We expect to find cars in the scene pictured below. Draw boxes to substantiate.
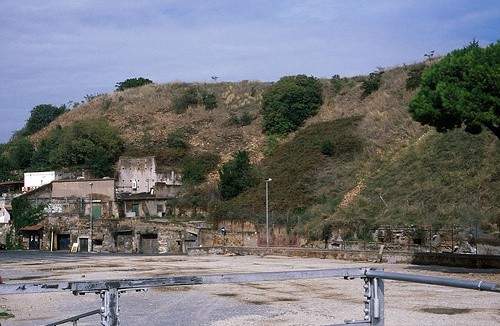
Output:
[76,177,84,180]
[103,177,110,179]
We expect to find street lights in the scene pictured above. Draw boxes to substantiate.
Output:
[89,183,94,251]
[265,178,272,249]
